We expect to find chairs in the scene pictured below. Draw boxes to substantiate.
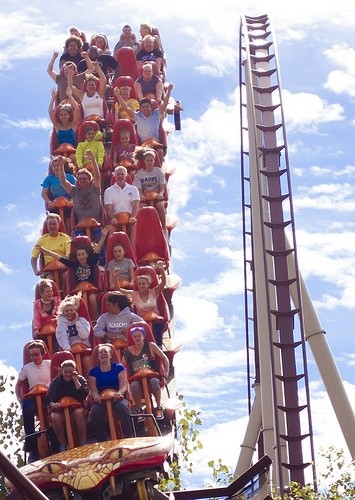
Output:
[13,30,181,425]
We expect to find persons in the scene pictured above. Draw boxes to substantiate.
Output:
[120,326,170,420]
[15,343,52,464]
[85,346,133,438]
[31,23,174,364]
[46,359,89,452]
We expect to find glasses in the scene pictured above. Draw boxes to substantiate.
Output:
[78,177,89,181]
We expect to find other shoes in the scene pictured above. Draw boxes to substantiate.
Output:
[155,406,164,420]
[136,408,145,422]
[162,229,168,241]
[28,451,37,463]
